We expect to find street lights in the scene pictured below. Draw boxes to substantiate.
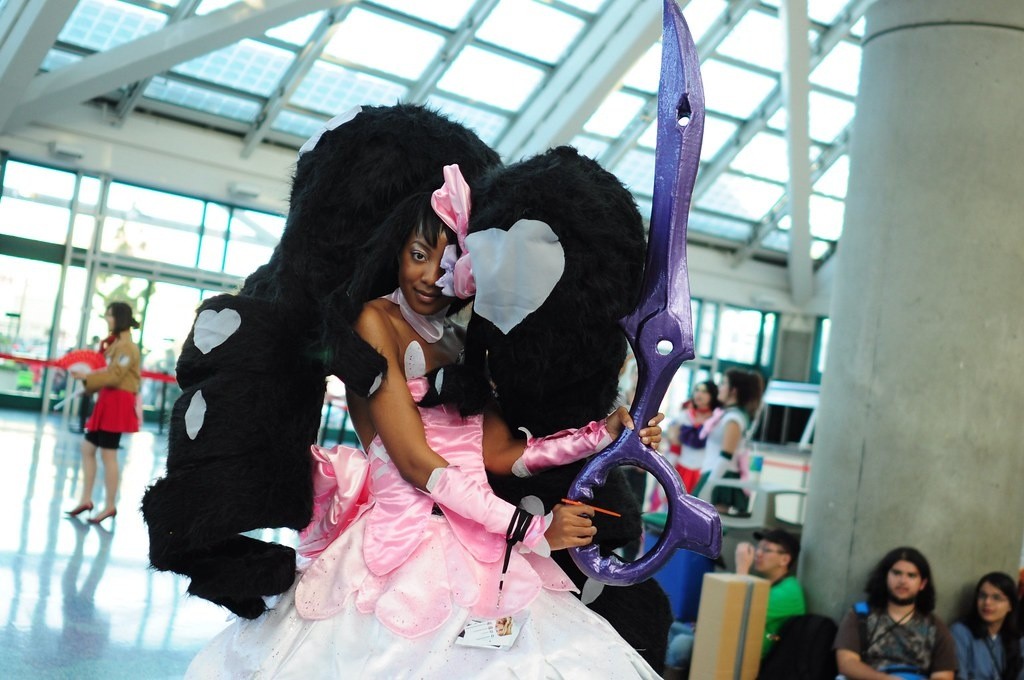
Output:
[5,312,20,344]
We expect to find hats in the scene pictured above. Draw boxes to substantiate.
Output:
[752,529,800,556]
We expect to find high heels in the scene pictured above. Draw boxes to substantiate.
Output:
[64,502,93,516]
[87,510,116,523]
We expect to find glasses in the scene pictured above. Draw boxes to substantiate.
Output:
[754,543,786,555]
[975,592,1008,604]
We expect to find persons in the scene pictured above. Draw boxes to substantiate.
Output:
[64,301,141,527]
[343,196,665,680]
[648,370,762,517]
[950,570,1024,680]
[667,526,807,673]
[835,547,958,680]
